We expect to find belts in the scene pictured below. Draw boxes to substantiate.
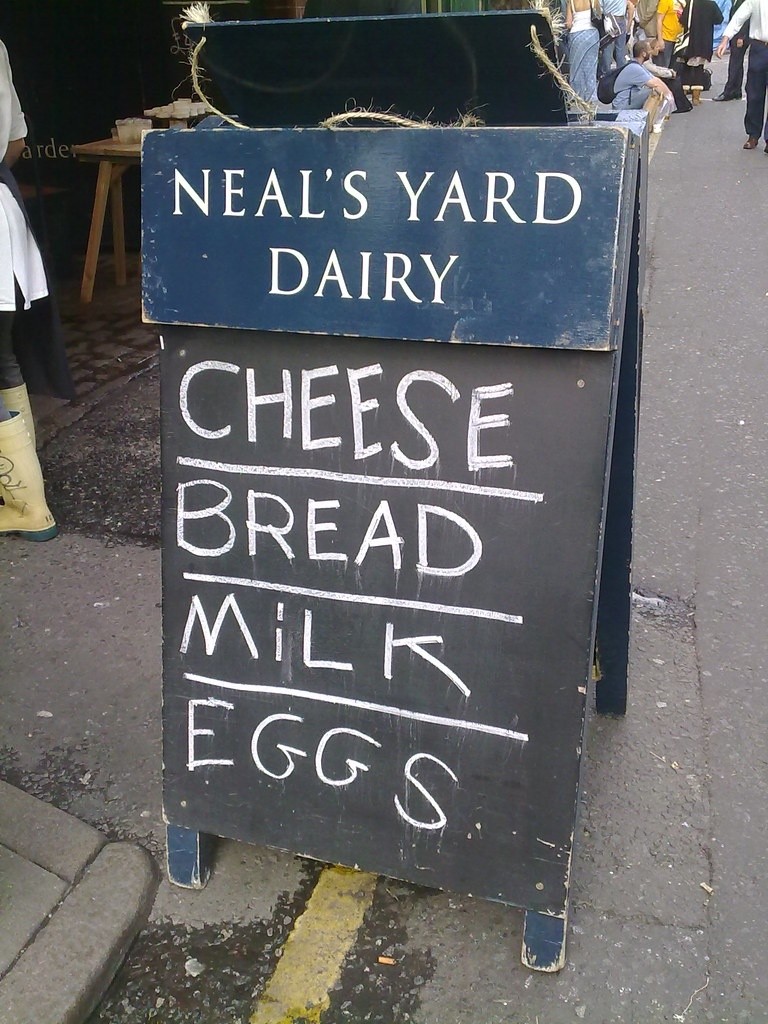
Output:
[750,39,768,48]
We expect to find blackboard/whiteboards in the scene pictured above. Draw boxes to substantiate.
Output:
[130,125,643,976]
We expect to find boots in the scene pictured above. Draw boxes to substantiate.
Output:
[690,85,704,106]
[0,384,60,542]
[683,85,691,96]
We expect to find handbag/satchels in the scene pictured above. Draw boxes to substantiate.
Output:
[589,0,604,29]
[641,88,664,134]
[594,11,622,51]
[672,31,691,60]
[675,62,713,91]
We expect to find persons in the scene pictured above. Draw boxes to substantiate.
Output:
[566,0,768,153]
[0,37,59,541]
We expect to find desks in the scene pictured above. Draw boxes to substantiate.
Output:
[71,137,142,304]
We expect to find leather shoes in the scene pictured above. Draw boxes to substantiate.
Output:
[743,138,759,149]
[712,90,742,102]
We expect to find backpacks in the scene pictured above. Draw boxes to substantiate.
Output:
[598,59,643,106]
[629,26,648,60]
[661,78,693,113]
